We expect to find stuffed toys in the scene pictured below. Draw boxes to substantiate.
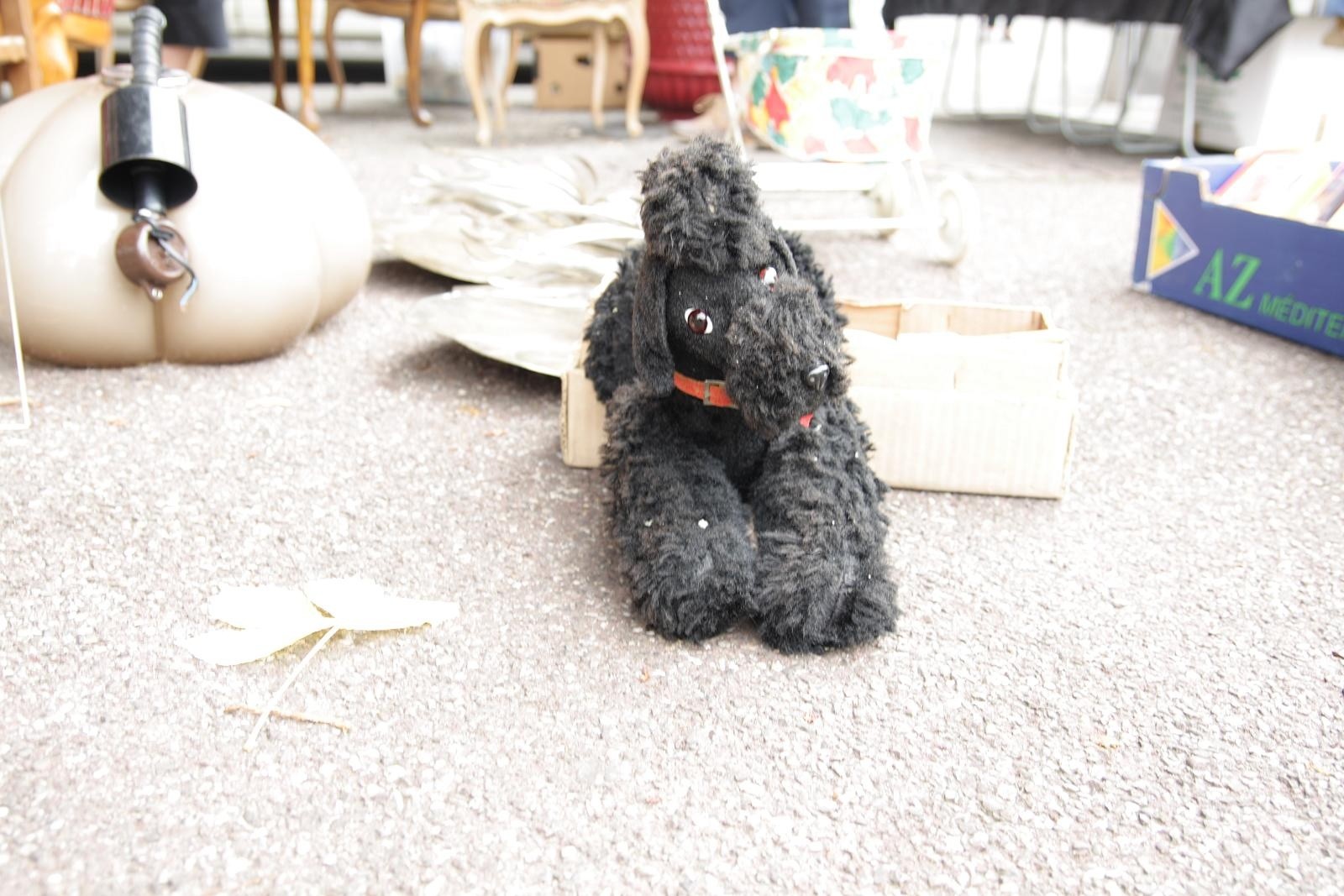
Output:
[577,132,897,657]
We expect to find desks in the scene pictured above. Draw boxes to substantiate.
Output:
[880,0,1292,162]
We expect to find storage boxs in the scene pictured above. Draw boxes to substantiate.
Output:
[561,294,1080,503]
[1128,147,1344,362]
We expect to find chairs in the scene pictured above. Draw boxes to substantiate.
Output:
[266,0,954,150]
[0,0,118,104]
